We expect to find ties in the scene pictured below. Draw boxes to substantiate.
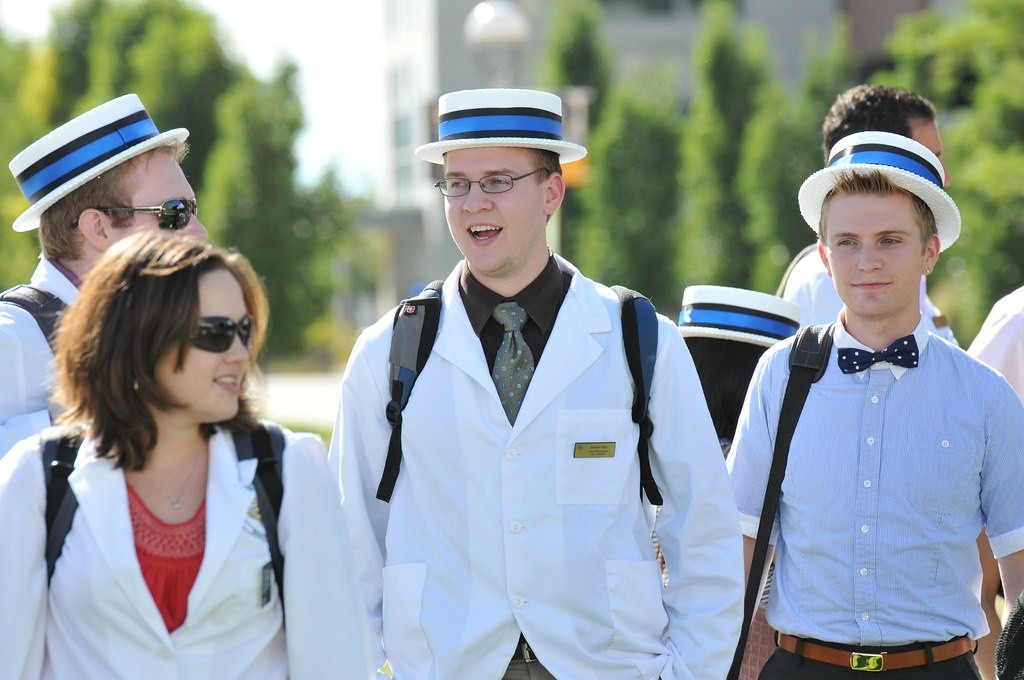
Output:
[492,302,536,427]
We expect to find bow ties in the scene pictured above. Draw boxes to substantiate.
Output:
[837,334,920,375]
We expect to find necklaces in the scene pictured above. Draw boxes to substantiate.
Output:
[134,444,206,508]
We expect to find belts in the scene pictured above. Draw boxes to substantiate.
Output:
[777,633,973,672]
[510,640,539,663]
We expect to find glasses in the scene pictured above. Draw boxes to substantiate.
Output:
[70,198,199,233]
[434,167,551,198]
[192,314,250,352]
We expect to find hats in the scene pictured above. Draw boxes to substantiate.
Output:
[8,93,191,233]
[671,285,805,350]
[794,131,962,255]
[413,88,587,166]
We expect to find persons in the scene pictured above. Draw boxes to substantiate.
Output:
[727,130,1023,677]
[775,86,957,348]
[0,230,363,678]
[0,92,210,463]
[962,279,1024,680]
[652,284,801,680]
[335,86,743,680]
